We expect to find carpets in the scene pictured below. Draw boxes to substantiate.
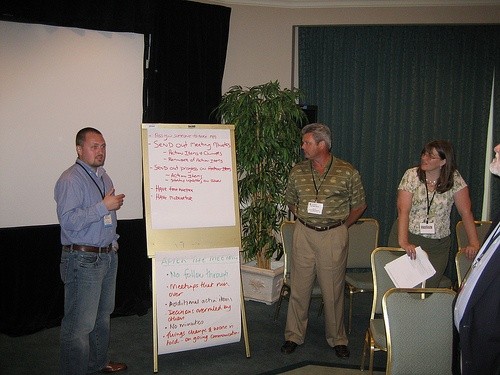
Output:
[258,361,386,375]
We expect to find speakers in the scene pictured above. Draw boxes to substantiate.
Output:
[293,105,318,129]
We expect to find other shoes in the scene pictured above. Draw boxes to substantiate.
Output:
[335,345,350,359]
[281,341,297,354]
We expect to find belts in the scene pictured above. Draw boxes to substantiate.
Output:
[64,244,112,253]
[296,216,346,231]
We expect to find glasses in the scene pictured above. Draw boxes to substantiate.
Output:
[422,151,440,159]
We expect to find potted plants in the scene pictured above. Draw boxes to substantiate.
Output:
[213,79,309,305]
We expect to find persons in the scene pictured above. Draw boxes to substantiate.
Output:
[452,144,500,375]
[281,123,365,358]
[388,139,480,298]
[54,127,128,375]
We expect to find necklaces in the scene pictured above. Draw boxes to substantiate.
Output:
[425,178,437,185]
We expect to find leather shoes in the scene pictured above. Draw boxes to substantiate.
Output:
[97,362,125,373]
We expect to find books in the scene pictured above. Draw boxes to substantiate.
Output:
[384,246,436,288]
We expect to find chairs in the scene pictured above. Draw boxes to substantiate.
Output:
[274,216,493,375]
